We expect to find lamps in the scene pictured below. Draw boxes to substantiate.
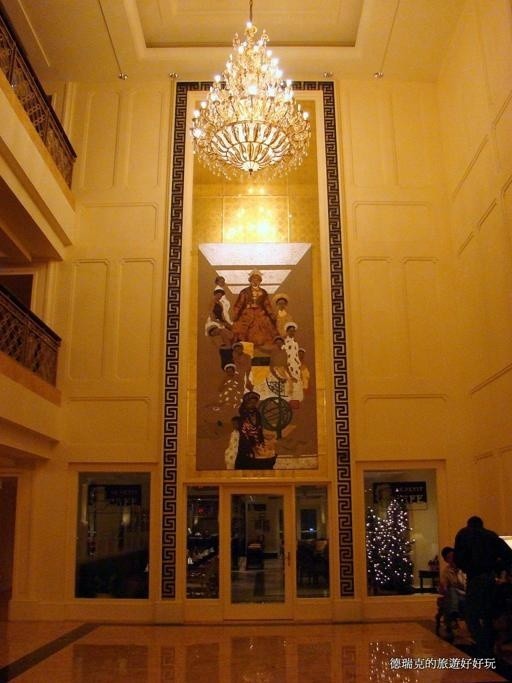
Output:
[187,1,313,185]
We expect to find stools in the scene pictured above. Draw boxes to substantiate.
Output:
[434,598,454,641]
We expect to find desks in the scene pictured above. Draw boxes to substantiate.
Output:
[418,568,440,594]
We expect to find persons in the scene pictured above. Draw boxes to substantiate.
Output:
[439,516,512,644]
[225,392,278,470]
[205,268,310,402]
